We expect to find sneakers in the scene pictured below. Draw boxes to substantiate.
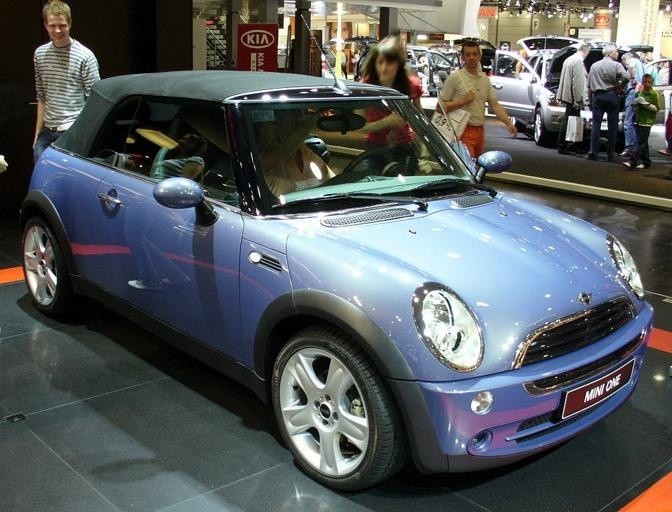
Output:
[556,148,672,171]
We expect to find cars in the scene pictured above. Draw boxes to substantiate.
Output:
[321,35,672,146]
[17,71,654,493]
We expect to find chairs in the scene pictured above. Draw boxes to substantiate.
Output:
[150,106,234,190]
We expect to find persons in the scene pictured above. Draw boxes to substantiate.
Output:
[361,36,422,176]
[435,42,517,160]
[255,110,331,199]
[33,1,102,169]
[556,42,672,170]
[125,126,208,292]
[327,41,348,79]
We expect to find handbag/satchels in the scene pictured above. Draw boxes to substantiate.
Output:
[429,108,471,147]
[564,115,583,143]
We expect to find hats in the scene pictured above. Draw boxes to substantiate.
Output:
[635,97,650,105]
